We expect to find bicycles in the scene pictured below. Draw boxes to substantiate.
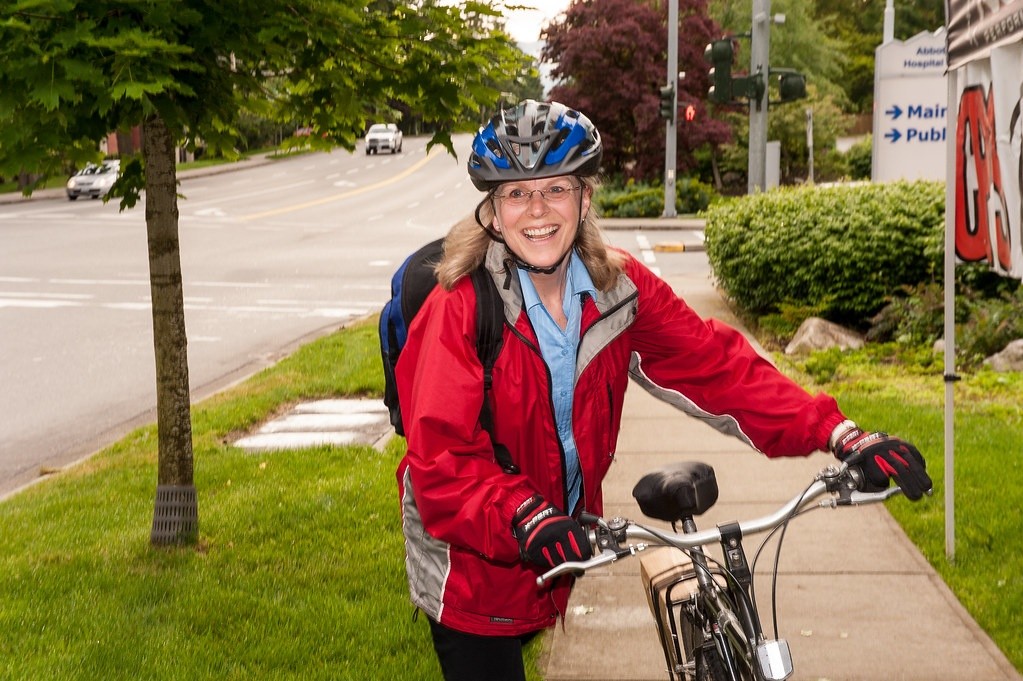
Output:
[536,454,934,681]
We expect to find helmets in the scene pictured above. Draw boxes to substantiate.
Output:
[467,100,603,192]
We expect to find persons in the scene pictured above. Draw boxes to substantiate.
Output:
[396,101,937,680]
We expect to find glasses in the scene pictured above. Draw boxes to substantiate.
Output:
[494,183,585,205]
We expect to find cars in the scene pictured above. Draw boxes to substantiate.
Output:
[365,121,403,155]
[68,160,141,202]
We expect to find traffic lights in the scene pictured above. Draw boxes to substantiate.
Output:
[658,83,676,126]
[702,39,734,104]
[683,103,697,122]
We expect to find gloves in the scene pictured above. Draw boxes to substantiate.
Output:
[512,494,593,576]
[835,426,933,502]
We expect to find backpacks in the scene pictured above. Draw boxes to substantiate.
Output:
[378,236,505,436]
